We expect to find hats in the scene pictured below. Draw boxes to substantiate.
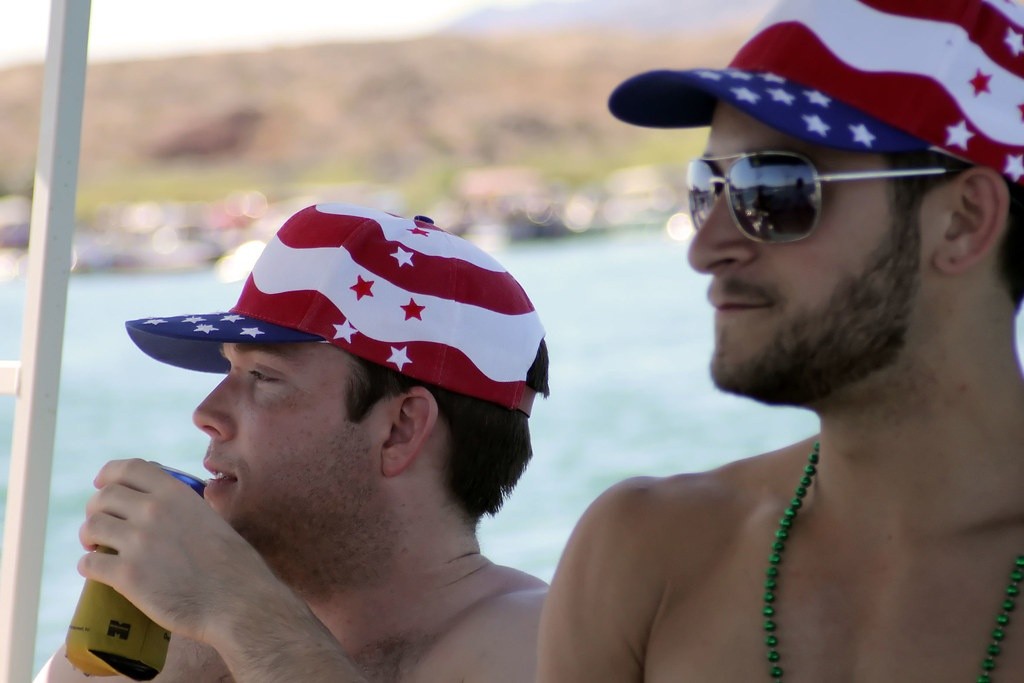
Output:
[125,203,545,417]
[608,0,1024,184]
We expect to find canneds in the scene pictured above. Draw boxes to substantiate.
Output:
[64,461,208,677]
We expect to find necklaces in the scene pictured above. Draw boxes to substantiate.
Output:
[761,441,1024,683]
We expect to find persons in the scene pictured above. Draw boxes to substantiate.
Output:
[28,199,554,683]
[533,0,1024,682]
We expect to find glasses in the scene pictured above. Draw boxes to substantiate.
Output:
[687,149,967,245]
